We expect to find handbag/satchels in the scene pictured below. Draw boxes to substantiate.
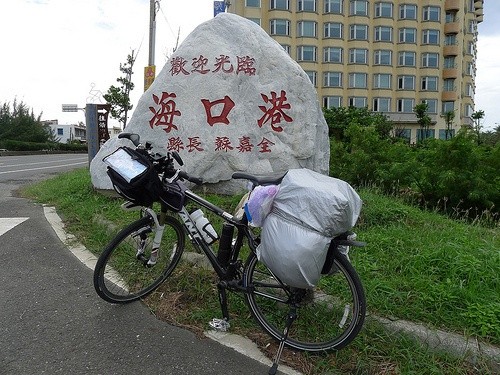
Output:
[244,183,279,226]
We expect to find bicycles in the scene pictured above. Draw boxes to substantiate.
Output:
[91,132,366,375]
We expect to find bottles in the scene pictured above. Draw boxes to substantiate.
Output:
[188,207,220,245]
[216,223,235,268]
